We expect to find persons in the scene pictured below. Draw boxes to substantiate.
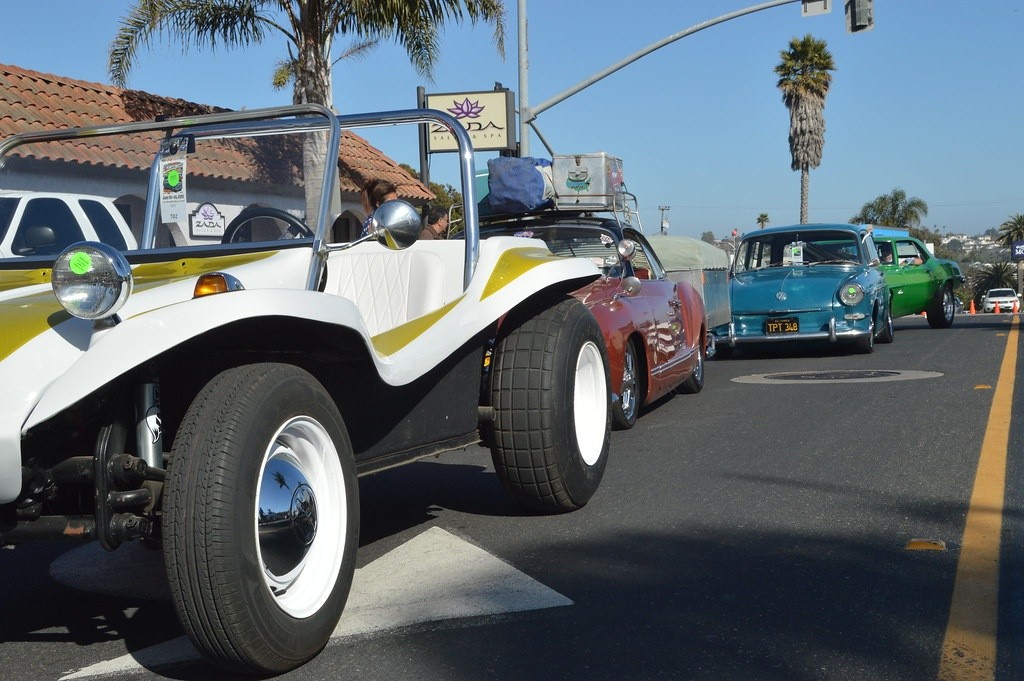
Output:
[418,207,448,240]
[881,245,923,266]
[360,178,397,240]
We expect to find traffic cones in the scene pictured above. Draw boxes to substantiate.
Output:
[1012,301,1019,313]
[969,298,976,314]
[994,300,1001,314]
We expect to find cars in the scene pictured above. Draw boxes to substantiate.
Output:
[712,223,894,359]
[981,288,1023,313]
[445,207,709,432]
[0,190,138,259]
[813,234,966,329]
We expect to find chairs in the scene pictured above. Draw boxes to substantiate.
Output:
[234,202,290,244]
[329,209,367,243]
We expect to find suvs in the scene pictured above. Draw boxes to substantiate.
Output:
[0,102,613,680]
